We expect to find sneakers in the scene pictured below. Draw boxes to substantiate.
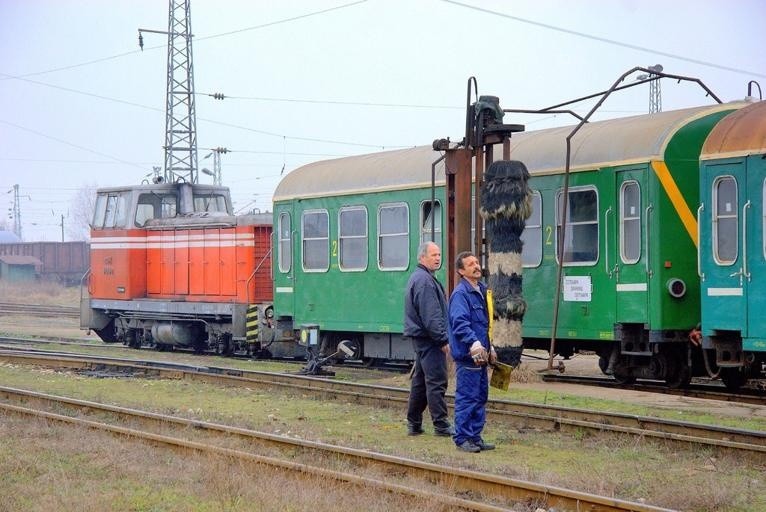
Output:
[475,439,496,451]
[408,428,426,436]
[433,427,456,436]
[459,439,480,453]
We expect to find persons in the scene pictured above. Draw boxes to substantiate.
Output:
[401,242,455,439]
[444,250,497,456]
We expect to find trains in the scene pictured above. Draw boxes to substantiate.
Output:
[0,241,90,287]
[74,64,766,391]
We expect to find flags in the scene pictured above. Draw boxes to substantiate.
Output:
[490,362,515,392]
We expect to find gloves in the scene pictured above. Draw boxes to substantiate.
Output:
[469,341,489,367]
[490,345,497,365]
